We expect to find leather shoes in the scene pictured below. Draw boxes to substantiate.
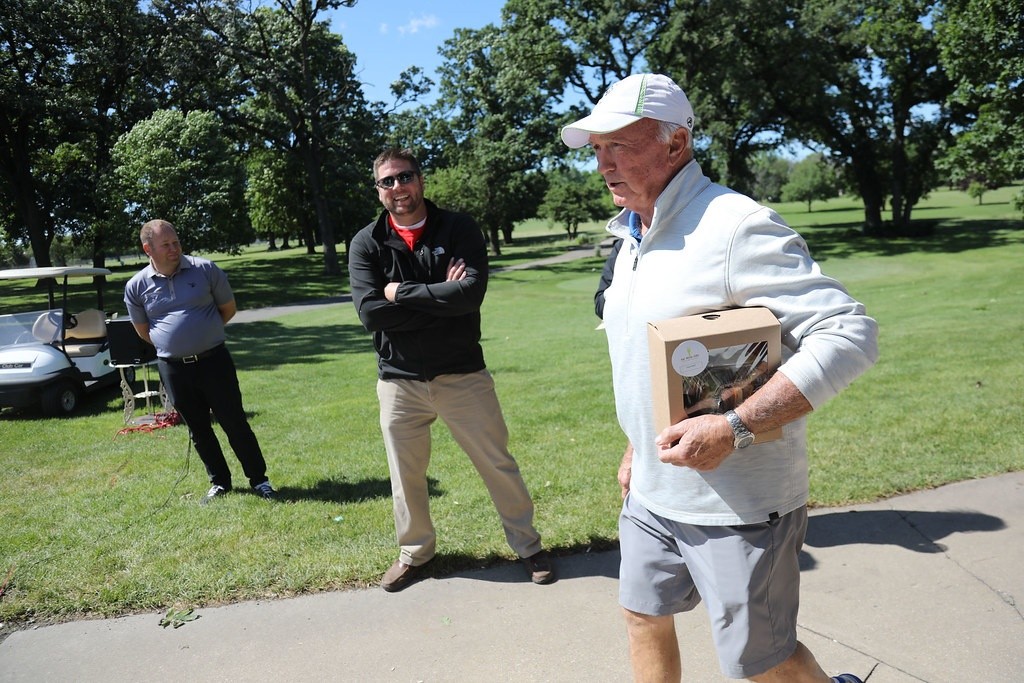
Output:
[519,553,554,584]
[380,561,417,592]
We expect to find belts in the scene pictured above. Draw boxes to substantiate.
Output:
[158,342,226,365]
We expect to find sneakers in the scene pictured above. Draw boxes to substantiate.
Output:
[200,485,233,506]
[252,484,282,503]
[831,673,863,683]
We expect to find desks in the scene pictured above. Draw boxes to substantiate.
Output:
[110,358,177,425]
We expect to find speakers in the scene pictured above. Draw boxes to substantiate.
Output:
[104,315,156,364]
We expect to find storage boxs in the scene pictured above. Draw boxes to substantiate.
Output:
[647,308,782,448]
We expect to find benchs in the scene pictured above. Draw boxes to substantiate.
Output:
[31,308,107,357]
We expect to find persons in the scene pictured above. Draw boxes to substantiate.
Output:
[562,73,879,683]
[347,150,554,593]
[123,220,277,504]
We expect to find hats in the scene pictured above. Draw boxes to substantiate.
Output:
[560,73,694,149]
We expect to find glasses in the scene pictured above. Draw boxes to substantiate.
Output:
[374,170,419,189]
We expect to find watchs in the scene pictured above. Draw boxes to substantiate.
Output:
[723,409,757,451]
